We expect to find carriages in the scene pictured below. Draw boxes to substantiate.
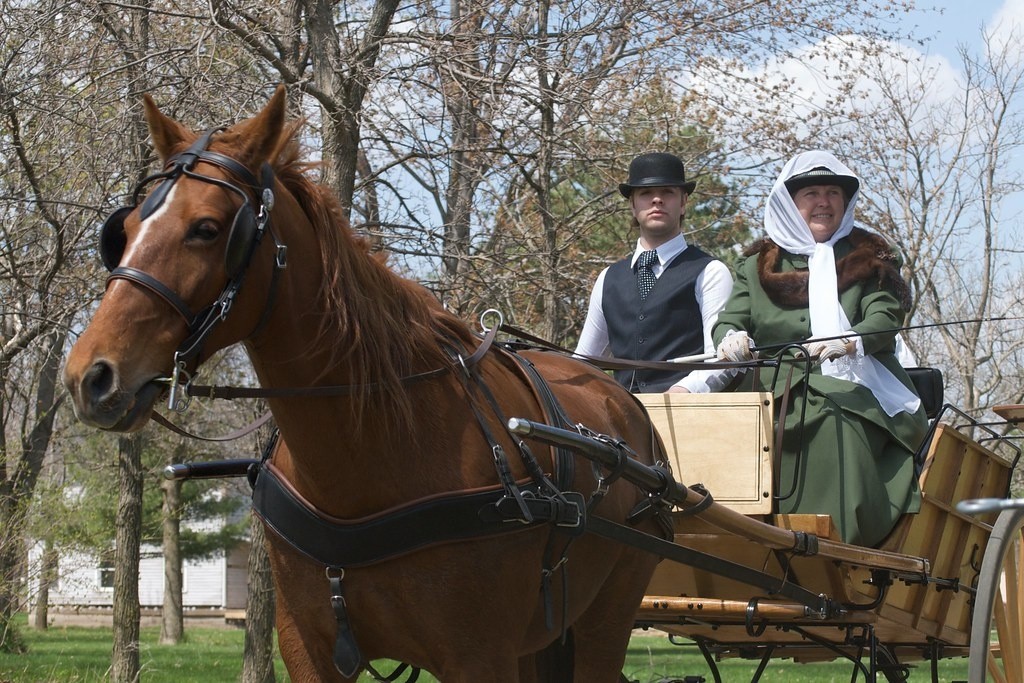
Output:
[61,83,1024,683]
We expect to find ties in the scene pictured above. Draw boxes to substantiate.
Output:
[636,249,658,302]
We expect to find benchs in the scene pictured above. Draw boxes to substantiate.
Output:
[853,366,946,554]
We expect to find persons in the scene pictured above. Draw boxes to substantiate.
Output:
[570,154,757,395]
[711,149,932,548]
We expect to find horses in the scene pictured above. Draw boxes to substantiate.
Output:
[61,81,673,683]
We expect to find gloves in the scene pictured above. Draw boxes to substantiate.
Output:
[716,329,759,377]
[794,335,846,371]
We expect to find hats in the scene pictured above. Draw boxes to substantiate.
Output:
[784,167,860,201]
[619,153,697,199]
